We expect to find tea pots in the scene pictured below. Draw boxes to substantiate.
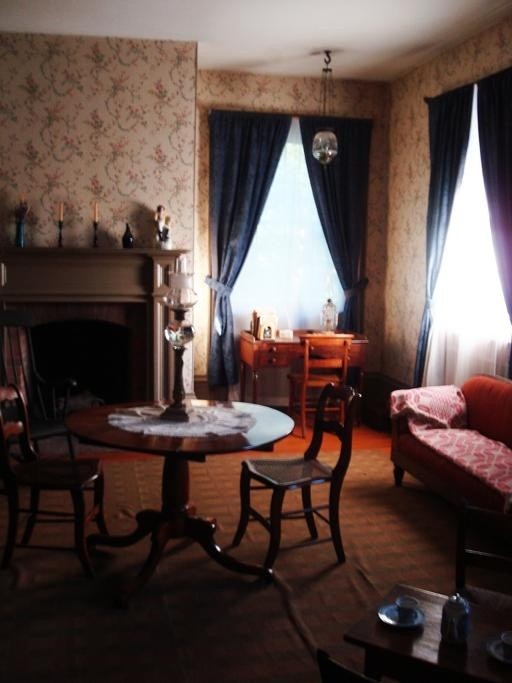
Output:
[440,593,474,638]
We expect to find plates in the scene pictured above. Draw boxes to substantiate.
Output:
[378,604,425,627]
[487,637,512,665]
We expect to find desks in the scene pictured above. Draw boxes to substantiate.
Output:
[64,398,296,610]
[240,328,370,430]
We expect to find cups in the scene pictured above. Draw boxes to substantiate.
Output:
[502,632,512,656]
[395,597,419,624]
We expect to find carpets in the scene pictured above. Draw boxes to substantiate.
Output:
[0,452,512,677]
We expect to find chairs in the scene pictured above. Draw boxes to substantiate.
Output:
[0,383,111,580]
[455,506,511,588]
[288,334,353,439]
[231,384,366,571]
[314,645,393,683]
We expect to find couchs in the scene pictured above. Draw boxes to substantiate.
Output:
[391,373,512,518]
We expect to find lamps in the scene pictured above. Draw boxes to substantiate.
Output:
[313,63,340,166]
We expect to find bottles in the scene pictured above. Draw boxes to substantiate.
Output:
[123,224,133,247]
